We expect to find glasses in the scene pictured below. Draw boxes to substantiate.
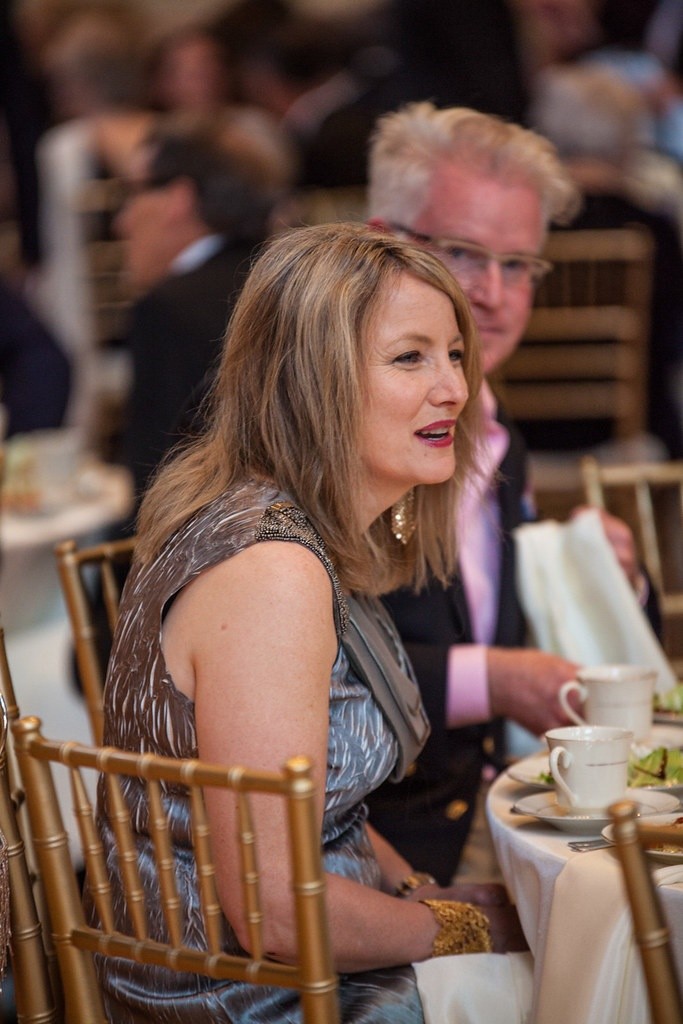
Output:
[389,223,554,281]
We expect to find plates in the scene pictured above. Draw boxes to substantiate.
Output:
[600,812,683,865]
[513,790,680,834]
[509,745,661,790]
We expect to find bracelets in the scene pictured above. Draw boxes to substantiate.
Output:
[397,872,437,898]
[419,898,493,958]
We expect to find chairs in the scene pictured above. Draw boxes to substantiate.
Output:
[0,538,342,1024]
[80,169,682,664]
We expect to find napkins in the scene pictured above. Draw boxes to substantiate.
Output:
[513,509,677,703]
[530,848,683,1024]
[412,952,535,1024]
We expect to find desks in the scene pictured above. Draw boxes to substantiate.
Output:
[487,725,683,969]
[0,446,130,871]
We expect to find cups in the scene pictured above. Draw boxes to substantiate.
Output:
[544,725,633,814]
[558,665,657,746]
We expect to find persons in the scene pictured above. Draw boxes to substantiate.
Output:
[0,0,683,699]
[82,224,533,1024]
[368,101,665,883]
